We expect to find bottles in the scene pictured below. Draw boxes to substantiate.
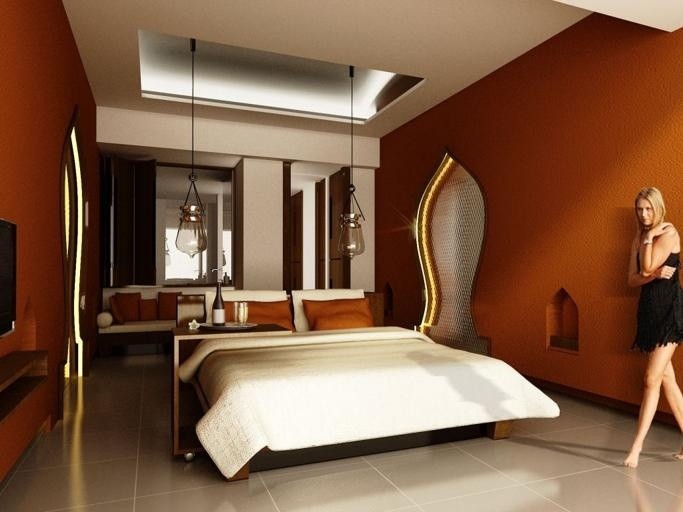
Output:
[212,282,226,326]
[224,272,229,286]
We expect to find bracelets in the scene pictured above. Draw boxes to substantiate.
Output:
[643,240,653,245]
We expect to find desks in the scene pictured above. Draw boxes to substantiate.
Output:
[170,323,293,460]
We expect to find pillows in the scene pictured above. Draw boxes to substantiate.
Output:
[157,291,181,320]
[115,291,141,321]
[138,298,157,322]
[223,300,295,333]
[302,298,375,332]
[109,295,125,325]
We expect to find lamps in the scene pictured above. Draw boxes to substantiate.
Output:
[176,37,209,258]
[338,65,366,258]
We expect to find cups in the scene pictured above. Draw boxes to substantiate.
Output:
[234,301,248,326]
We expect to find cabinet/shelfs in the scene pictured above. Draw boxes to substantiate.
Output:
[0,349,58,484]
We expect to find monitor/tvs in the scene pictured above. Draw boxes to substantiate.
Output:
[0,216,17,338]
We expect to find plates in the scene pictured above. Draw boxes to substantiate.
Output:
[200,322,258,330]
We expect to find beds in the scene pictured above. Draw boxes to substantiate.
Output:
[176,293,560,483]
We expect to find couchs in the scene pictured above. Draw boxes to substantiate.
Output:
[97,286,235,357]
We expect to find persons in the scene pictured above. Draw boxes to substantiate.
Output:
[622,187,683,468]
